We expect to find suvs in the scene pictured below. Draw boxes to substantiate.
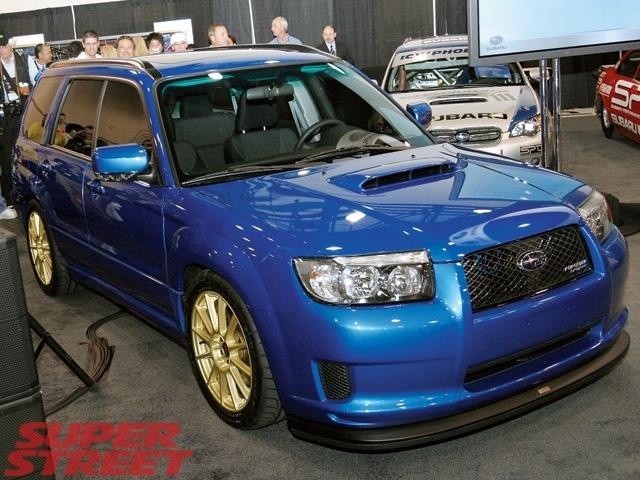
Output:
[10,40,631,456]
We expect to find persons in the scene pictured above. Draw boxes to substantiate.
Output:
[268,16,302,45]
[316,25,355,65]
[0,23,237,221]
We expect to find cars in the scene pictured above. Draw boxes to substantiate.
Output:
[375,34,553,169]
[593,48,640,149]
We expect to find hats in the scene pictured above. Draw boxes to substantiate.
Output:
[0,29,12,46]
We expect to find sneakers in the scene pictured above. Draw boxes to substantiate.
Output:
[0,204,20,221]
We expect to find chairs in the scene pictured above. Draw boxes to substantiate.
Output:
[177,94,236,173]
[222,89,302,167]
[158,107,209,181]
